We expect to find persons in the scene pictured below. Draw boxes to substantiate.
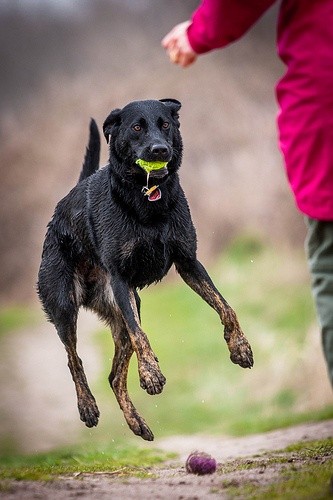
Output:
[161,1,333,386]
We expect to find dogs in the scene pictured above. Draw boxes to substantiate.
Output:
[33,97,254,441]
